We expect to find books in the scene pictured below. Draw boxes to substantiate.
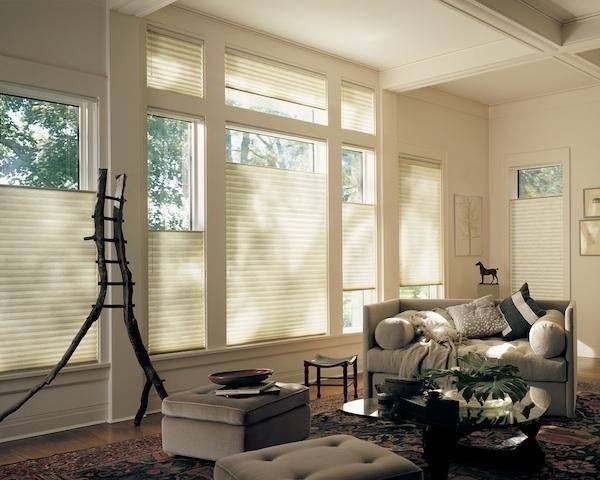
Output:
[216,383,280,396]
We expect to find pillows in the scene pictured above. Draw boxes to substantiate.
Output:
[529,308,565,359]
[446,295,508,338]
[408,306,462,344]
[495,283,545,341]
[373,309,420,350]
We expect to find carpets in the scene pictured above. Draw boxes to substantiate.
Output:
[1,381,600,480]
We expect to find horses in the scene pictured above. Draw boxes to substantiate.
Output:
[476,261,499,284]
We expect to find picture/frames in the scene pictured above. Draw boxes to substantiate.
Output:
[583,188,600,218]
[453,192,484,257]
[579,220,600,256]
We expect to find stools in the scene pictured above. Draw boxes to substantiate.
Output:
[162,381,310,463]
[214,435,424,480]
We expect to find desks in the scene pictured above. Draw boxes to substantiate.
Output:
[304,353,357,403]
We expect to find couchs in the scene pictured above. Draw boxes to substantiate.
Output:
[363,299,576,420]
